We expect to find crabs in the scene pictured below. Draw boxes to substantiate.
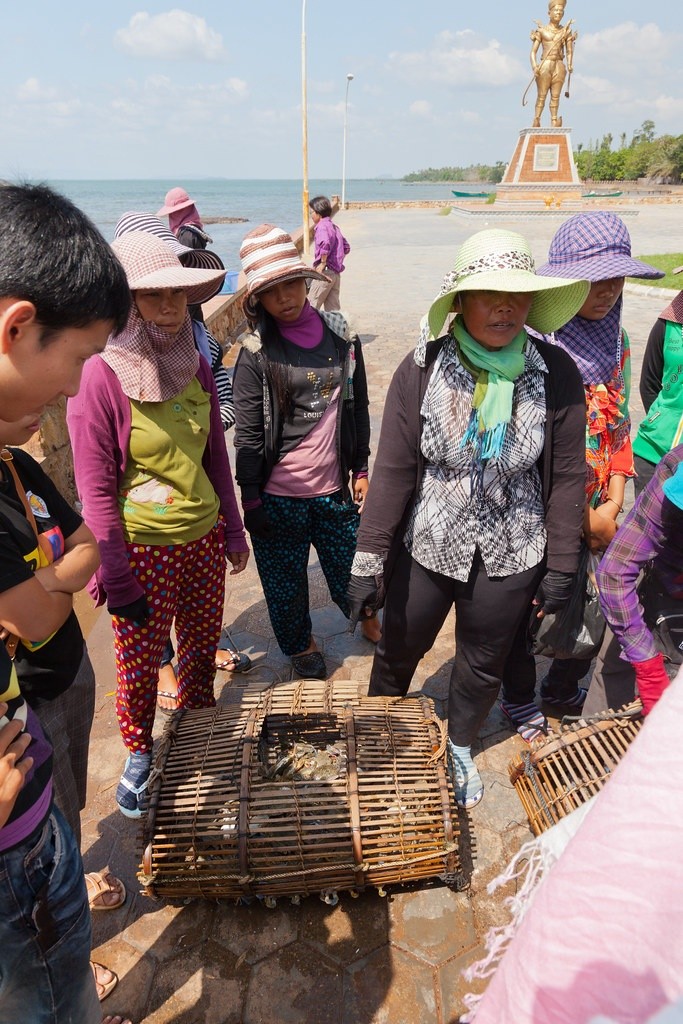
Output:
[184,740,434,907]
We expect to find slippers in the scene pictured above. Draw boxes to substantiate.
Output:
[216,647,251,673]
[361,627,384,645]
[445,752,484,810]
[156,690,180,716]
[500,702,555,745]
[290,636,327,680]
[117,756,150,820]
[89,960,118,1002]
[542,687,588,714]
[84,871,127,912]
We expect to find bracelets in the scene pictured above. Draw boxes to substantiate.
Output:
[605,497,624,513]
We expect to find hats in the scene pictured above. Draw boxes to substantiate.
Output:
[155,186,196,218]
[413,227,591,368]
[95,231,226,290]
[239,223,332,324]
[658,265,683,325]
[535,210,666,283]
[114,211,225,270]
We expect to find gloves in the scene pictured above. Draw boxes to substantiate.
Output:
[107,594,148,626]
[632,652,670,715]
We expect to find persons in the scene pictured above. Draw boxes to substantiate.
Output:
[348,227,586,809]
[66,185,251,821]
[306,196,351,313]
[0,178,132,1024]
[233,224,384,678]
[497,210,683,747]
[530,0,575,127]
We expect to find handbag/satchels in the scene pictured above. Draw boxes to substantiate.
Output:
[528,547,607,661]
